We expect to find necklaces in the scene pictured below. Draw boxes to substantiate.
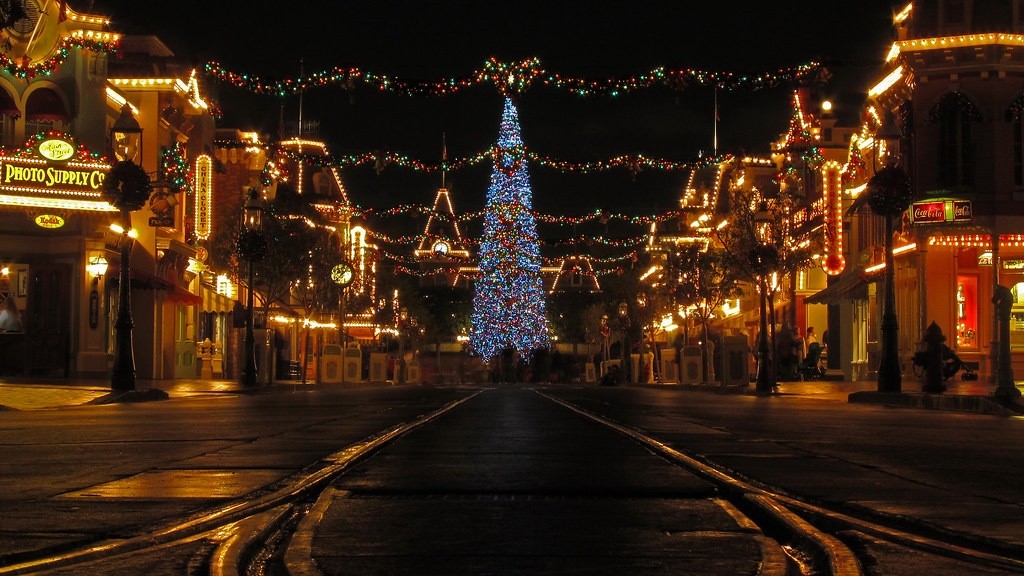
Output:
[806,327,816,354]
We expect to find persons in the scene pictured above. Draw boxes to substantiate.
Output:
[792,326,806,376]
[550,371,558,382]
[823,331,827,350]
[593,350,603,383]
[500,342,515,384]
[603,364,621,386]
[397,355,406,383]
[386,355,395,380]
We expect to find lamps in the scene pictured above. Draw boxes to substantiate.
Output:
[93,255,108,280]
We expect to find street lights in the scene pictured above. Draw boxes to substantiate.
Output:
[108,102,144,393]
[620,299,631,383]
[242,194,263,387]
[754,200,775,396]
[870,103,904,402]
[601,314,608,380]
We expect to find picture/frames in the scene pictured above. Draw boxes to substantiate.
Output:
[17,269,26,297]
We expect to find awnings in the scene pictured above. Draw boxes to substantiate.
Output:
[200,285,236,313]
[744,300,792,327]
[253,307,296,317]
[803,269,864,304]
[106,262,202,304]
[155,237,199,259]
[711,308,757,328]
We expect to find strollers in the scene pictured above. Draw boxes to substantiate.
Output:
[800,342,826,380]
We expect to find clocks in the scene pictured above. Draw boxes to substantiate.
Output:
[330,264,352,284]
